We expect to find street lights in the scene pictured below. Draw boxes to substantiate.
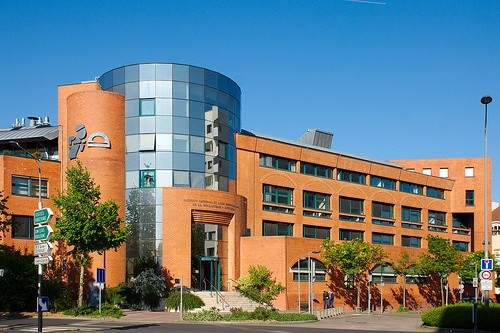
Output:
[308,250,322,315]
[470,262,477,302]
[481,95,494,307]
[10,140,43,333]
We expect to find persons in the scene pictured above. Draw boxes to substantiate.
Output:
[323,290,334,309]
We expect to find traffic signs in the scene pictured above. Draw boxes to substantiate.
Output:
[34,256,53,266]
[34,225,53,241]
[34,242,53,255]
[34,208,53,224]
